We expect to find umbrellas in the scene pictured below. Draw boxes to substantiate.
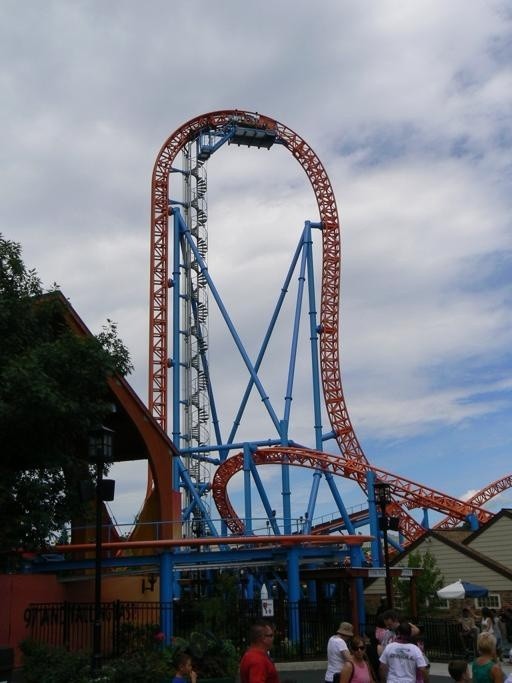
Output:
[436,578,490,611]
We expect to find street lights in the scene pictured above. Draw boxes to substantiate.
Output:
[86,416,116,677]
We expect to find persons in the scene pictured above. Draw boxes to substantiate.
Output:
[448,600,511,682]
[324,611,431,682]
[239,622,279,682]
[169,652,197,682]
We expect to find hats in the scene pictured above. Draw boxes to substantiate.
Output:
[337,622,354,636]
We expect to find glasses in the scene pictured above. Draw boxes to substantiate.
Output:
[354,646,365,651]
[266,634,273,637]
